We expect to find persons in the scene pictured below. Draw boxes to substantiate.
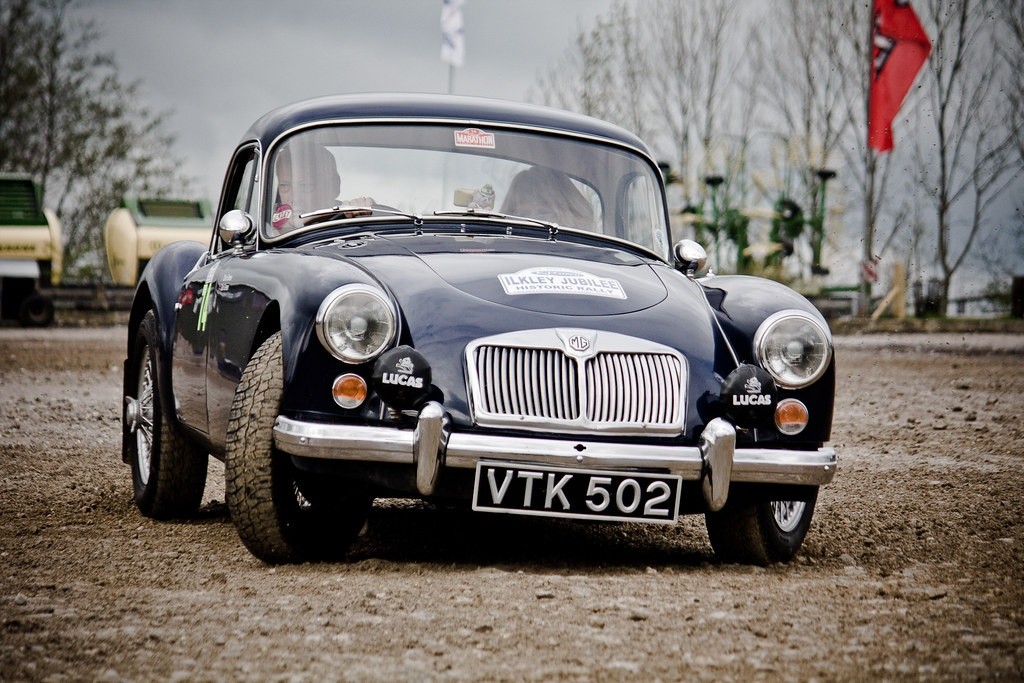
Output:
[276,143,376,234]
[499,165,594,234]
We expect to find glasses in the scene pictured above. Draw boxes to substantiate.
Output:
[278,178,323,194]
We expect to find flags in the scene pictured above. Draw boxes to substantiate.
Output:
[870,1,932,153]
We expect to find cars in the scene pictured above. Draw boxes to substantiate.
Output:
[119,95,846,571]
